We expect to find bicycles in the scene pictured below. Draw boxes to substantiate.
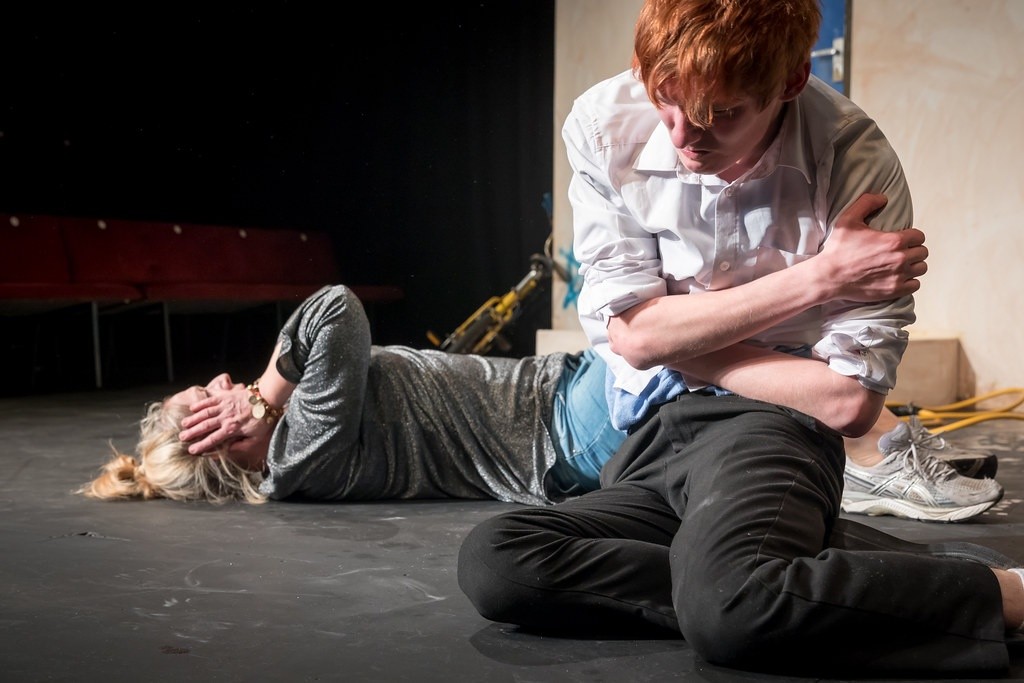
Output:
[423,229,573,355]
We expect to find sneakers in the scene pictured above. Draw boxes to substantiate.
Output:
[841,415,1004,521]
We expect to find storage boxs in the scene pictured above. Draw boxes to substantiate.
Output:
[535,329,589,355]
[886,338,977,412]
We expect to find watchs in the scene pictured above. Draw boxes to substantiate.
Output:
[247,381,285,420]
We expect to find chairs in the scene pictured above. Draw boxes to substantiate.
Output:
[62,217,147,389]
[138,220,250,384]
[222,225,316,333]
[279,230,396,305]
[0,213,74,316]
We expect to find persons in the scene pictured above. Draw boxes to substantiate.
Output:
[67,281,1006,526]
[454,0,1024,680]
